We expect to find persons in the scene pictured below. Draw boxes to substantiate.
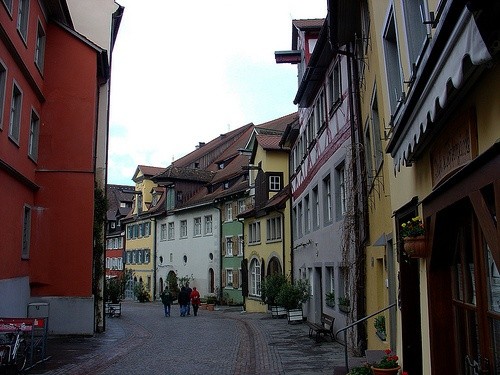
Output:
[178,282,201,317]
[159,286,173,317]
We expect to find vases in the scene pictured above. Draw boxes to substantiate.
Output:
[403,237,429,259]
[371,367,401,375]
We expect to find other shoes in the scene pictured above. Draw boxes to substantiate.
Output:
[187,312,190,315]
[180,313,185,316]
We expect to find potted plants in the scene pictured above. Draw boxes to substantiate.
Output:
[277,280,313,323]
[325,289,334,307]
[200,298,208,310]
[376,316,387,340]
[338,296,351,313]
[207,297,215,311]
[259,270,289,319]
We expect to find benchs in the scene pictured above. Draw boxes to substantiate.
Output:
[308,313,336,344]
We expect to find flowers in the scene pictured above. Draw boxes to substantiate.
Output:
[373,349,398,369]
[401,217,425,237]
